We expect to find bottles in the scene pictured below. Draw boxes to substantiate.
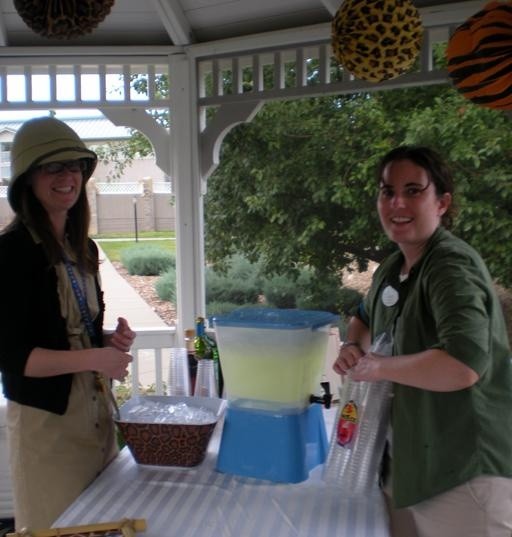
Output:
[180,318,223,397]
[326,325,341,364]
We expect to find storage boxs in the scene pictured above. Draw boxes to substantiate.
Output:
[212,302,344,416]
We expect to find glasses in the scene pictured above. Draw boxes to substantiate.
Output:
[39,160,88,174]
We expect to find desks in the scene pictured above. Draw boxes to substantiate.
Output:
[50,417,391,537]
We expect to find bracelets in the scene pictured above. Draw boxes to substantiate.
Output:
[342,341,361,346]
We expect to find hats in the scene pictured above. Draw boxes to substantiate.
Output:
[6,117,97,210]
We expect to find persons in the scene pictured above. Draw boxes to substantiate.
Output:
[333,144,512,537]
[0,116,137,532]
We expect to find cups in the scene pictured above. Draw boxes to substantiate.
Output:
[321,366,393,502]
[165,349,222,397]
